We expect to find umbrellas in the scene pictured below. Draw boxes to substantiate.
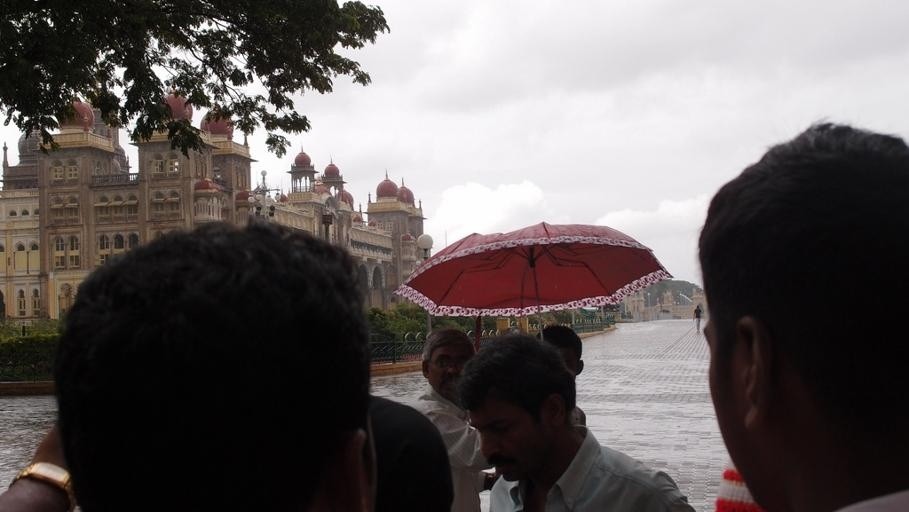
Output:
[392,222,674,339]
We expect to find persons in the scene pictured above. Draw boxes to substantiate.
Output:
[1,214,377,510]
[693,305,702,333]
[413,327,496,510]
[368,387,454,512]
[537,324,585,426]
[700,120,909,509]
[458,331,697,510]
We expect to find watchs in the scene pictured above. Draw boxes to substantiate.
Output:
[8,461,81,510]
